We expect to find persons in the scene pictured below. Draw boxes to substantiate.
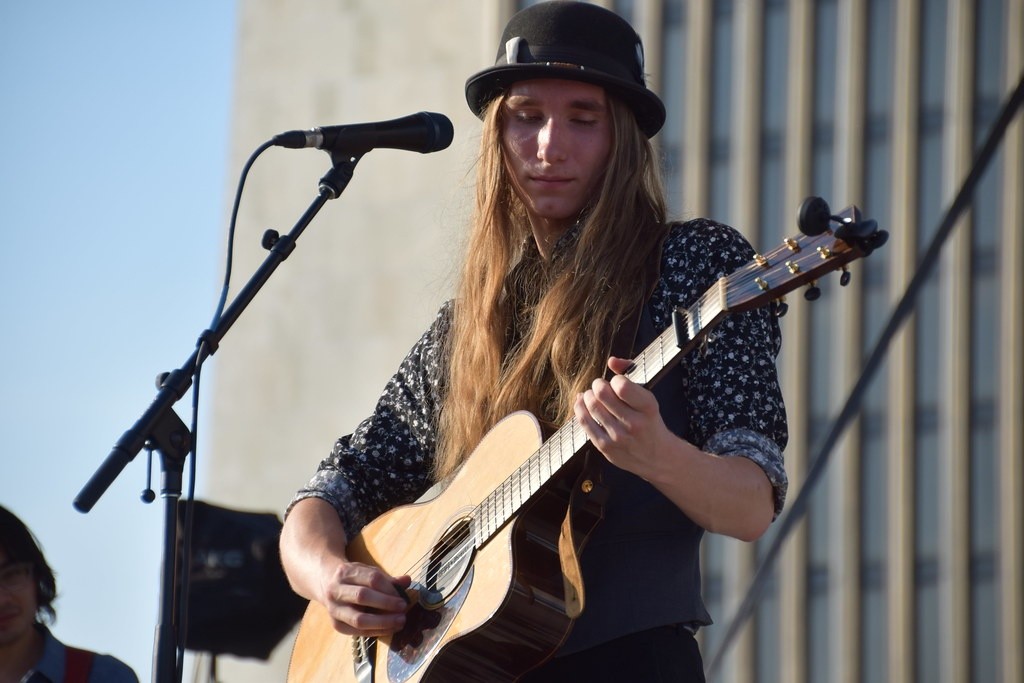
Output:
[279,2,789,683]
[0,504,140,683]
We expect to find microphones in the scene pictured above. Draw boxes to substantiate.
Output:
[270,111,454,154]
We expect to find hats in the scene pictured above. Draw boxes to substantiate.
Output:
[465,1,666,139]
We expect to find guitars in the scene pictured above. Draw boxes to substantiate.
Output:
[283,194,889,682]
